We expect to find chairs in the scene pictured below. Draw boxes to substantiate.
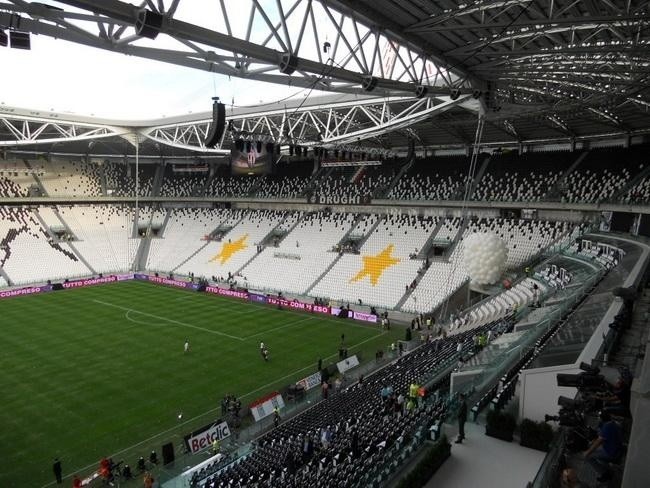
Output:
[190,311,522,487]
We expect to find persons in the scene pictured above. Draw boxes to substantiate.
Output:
[1,159,650,488]
